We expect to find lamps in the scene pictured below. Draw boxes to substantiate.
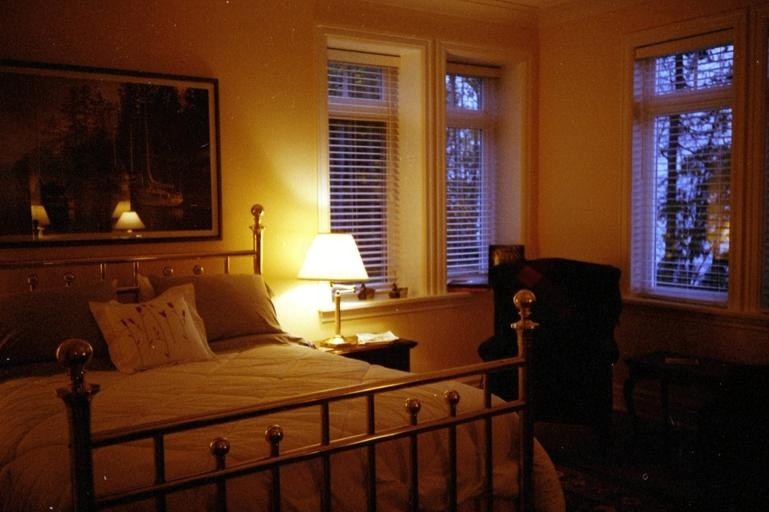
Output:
[295,230,372,347]
[109,200,147,233]
[31,205,49,239]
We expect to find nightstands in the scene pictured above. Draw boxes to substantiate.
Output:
[312,332,416,373]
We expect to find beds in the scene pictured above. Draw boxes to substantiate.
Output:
[2,205,566,511]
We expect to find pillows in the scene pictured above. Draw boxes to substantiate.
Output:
[2,271,288,379]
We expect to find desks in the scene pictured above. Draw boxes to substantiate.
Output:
[623,350,744,444]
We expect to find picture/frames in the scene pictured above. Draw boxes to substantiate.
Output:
[2,59,223,251]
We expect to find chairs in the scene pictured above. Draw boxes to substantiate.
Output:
[479,259,621,437]
